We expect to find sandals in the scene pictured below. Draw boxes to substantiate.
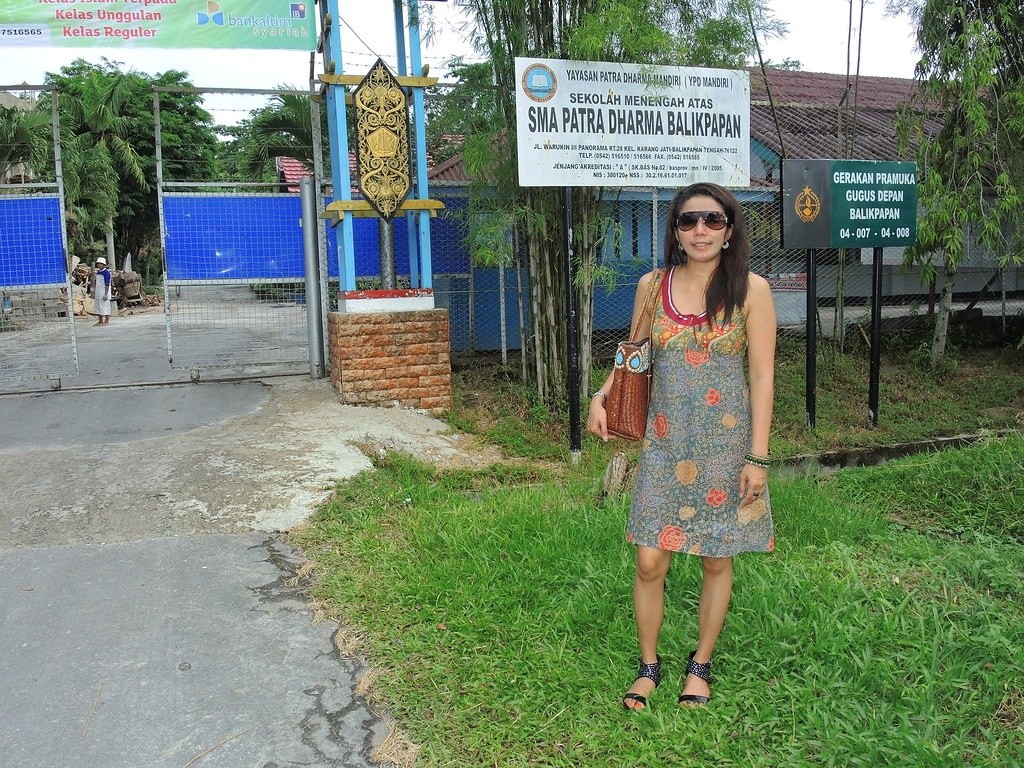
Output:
[622,653,662,710]
[678,650,713,708]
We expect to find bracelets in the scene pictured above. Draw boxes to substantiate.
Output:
[593,391,607,403]
[745,454,771,468]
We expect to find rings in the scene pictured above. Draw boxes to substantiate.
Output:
[754,493,759,496]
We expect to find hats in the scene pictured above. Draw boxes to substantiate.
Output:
[95,257,106,266]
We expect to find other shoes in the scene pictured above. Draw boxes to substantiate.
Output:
[91,322,103,328]
[103,319,109,326]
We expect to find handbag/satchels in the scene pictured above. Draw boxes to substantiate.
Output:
[606,268,663,441]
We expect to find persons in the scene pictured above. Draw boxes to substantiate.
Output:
[92,257,111,326]
[587,182,775,710]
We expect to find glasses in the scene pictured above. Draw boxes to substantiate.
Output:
[674,211,728,232]
